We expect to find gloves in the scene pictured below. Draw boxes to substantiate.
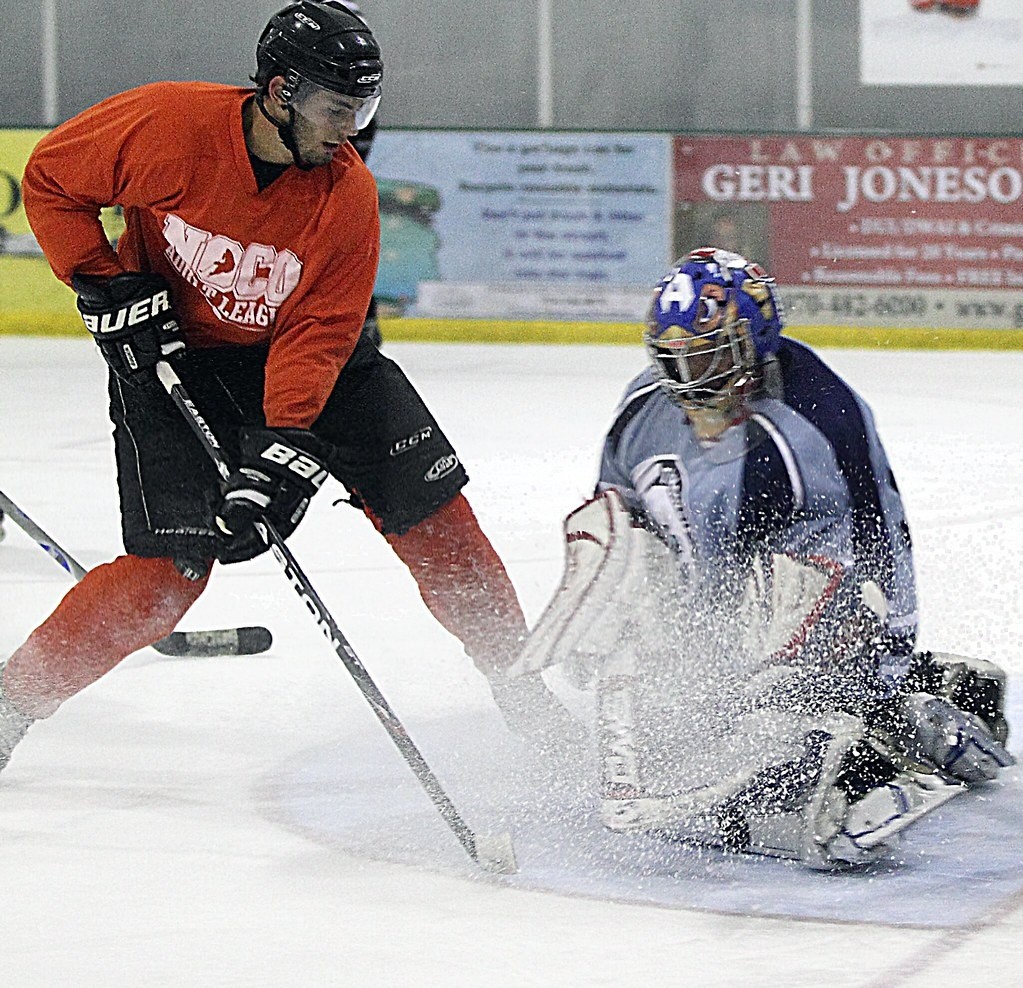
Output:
[67,270,187,396]
[213,424,337,565]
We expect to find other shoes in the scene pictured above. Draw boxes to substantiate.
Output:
[490,676,591,758]
[0,661,34,773]
[900,690,1016,783]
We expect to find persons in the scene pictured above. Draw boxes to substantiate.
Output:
[0,0,589,771]
[504,244,1023,872]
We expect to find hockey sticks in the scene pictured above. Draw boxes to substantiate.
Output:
[154,361,519,876]
[1,490,272,659]
[598,645,757,836]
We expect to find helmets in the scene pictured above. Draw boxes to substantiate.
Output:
[256,0,384,100]
[644,245,787,439]
